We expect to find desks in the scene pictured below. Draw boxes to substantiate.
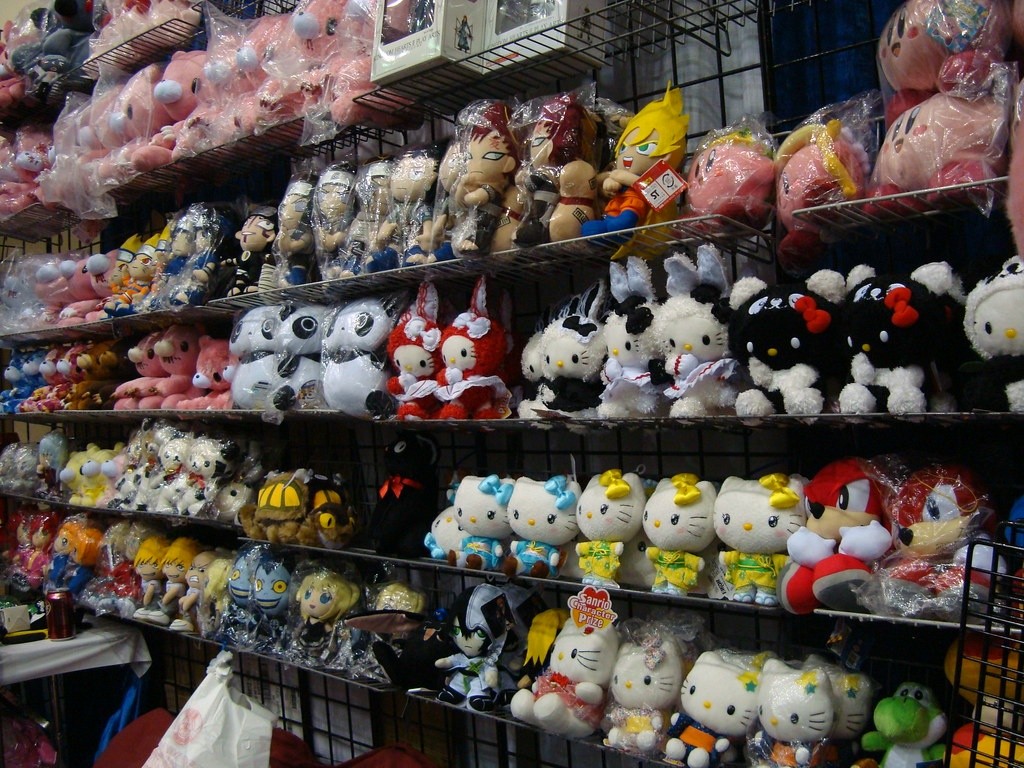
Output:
[0,612,151,768]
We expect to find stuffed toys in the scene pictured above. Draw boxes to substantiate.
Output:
[0,0,1024,768]
[321,290,409,419]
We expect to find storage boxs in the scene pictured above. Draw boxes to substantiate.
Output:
[370,0,487,115]
[483,0,608,90]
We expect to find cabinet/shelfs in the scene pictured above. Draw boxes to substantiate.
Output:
[0,0,1024,768]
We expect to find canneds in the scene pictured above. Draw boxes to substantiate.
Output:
[46,587,76,641]
